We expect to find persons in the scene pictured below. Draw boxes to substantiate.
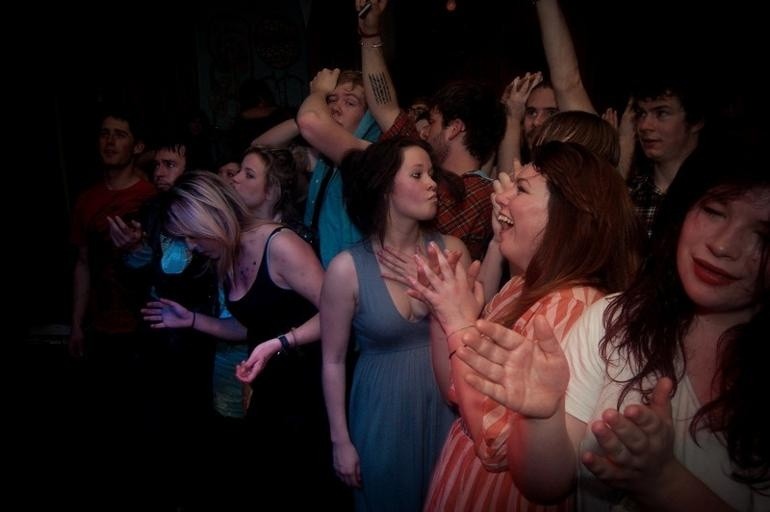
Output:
[456,130,769,512]
[319,137,473,510]
[55,1,746,447]
[406,141,644,512]
[139,171,357,511]
